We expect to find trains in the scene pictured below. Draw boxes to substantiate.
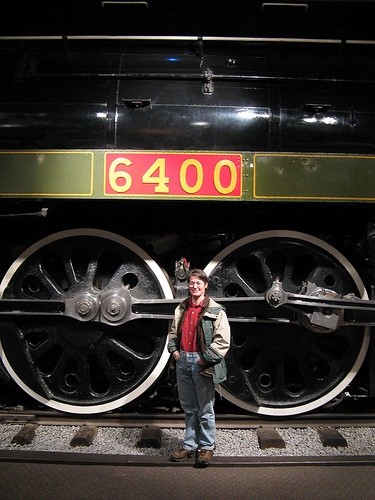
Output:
[1,0,375,419]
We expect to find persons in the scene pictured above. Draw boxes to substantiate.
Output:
[167,270,230,465]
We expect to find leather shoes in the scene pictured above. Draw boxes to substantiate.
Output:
[170,446,217,465]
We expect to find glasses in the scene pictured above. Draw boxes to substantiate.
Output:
[188,282,205,287]
